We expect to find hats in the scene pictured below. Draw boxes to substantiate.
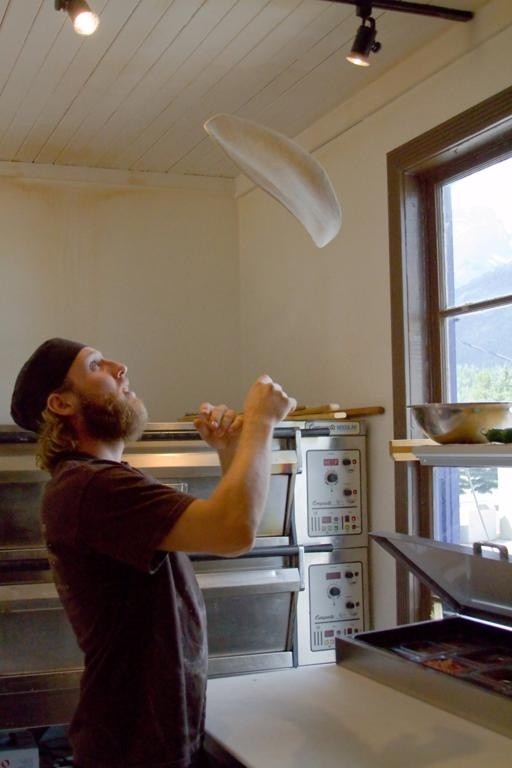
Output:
[11,337,86,432]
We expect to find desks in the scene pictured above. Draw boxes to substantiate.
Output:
[200,663,512,768]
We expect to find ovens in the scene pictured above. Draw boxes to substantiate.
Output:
[1,416,370,746]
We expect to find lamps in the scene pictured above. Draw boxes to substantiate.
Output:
[56,0,99,35]
[346,4,380,69]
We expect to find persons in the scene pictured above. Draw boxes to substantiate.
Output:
[9,337,298,766]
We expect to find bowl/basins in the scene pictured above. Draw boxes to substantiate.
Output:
[405,399,509,446]
